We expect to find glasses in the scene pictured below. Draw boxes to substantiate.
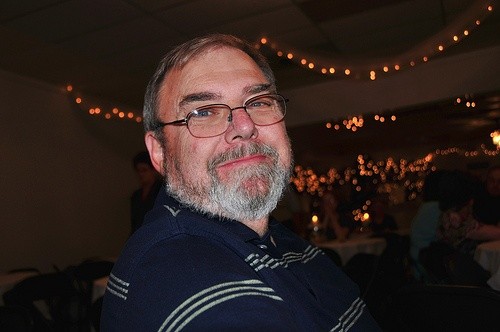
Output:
[153,93,290,139]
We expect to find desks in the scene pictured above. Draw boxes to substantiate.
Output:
[475,240,500,293]
[0,271,53,320]
[319,237,388,266]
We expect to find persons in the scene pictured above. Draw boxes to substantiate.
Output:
[128,152,165,232]
[371,199,401,237]
[438,186,500,291]
[97,32,386,332]
